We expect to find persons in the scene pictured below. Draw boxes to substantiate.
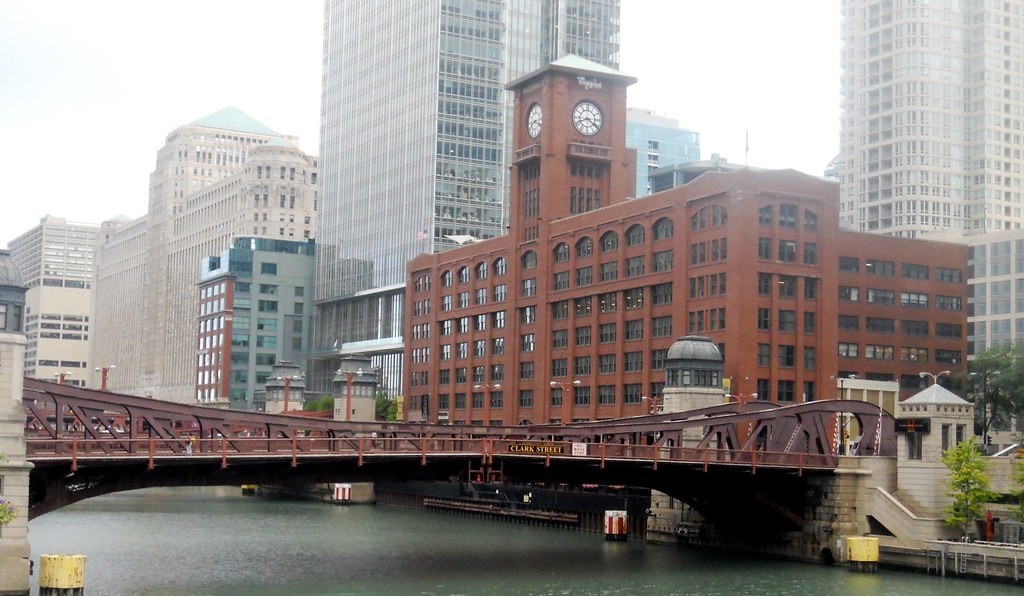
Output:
[187,433,196,454]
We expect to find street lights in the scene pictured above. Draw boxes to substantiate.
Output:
[96,365,116,390]
[641,396,671,414]
[276,376,298,414]
[54,372,72,383]
[830,374,855,455]
[725,393,758,414]
[337,370,363,420]
[970,371,1001,456]
[549,380,581,426]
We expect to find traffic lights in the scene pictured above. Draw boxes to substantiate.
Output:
[987,435,992,447]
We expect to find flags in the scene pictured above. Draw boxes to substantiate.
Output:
[418,230,428,239]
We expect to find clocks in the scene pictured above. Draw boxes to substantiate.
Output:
[572,102,603,136]
[527,104,543,139]
